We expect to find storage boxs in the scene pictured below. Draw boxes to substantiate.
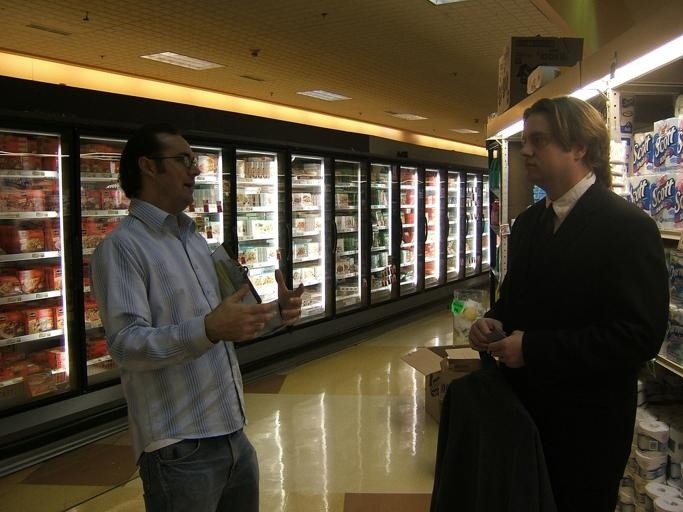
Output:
[496,37,584,115]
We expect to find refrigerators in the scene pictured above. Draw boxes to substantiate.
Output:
[398,161,444,298]
[233,145,329,345]
[333,152,398,319]
[183,141,229,374]
[444,164,478,287]
[480,170,489,276]
[1,74,134,416]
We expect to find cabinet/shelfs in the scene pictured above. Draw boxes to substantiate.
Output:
[0,74,502,477]
[607,85,683,380]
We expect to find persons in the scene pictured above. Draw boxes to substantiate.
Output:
[431,96,670,511]
[88,124,305,511]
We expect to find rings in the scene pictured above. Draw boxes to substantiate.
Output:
[254,321,265,331]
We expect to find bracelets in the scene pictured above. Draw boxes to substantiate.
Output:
[278,303,283,321]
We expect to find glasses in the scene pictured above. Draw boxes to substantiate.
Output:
[151,155,197,167]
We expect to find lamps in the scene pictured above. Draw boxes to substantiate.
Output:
[486,0,683,140]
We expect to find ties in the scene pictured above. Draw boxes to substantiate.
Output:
[540,204,554,239]
